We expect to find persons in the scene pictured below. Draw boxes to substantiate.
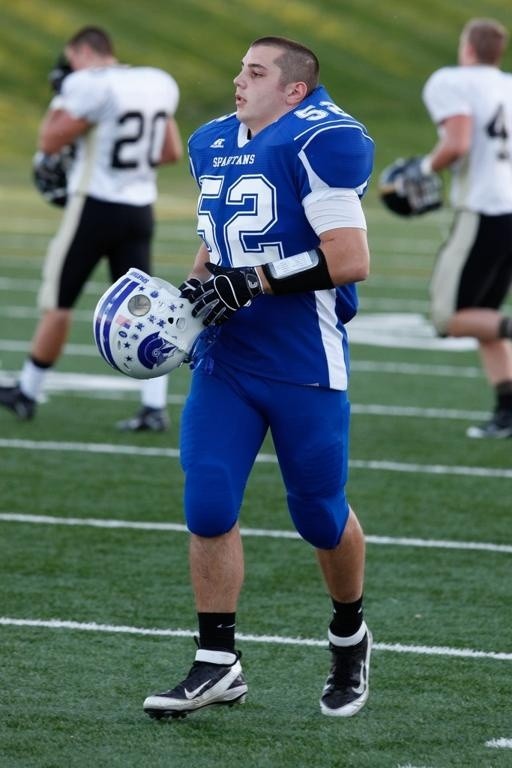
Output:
[396,16,512,440]
[144,36,376,717]
[1,25,183,431]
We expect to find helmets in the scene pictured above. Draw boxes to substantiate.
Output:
[377,156,443,216]
[32,142,76,207]
[92,267,223,379]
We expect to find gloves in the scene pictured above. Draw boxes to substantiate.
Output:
[176,262,264,327]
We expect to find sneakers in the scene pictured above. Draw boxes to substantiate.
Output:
[466,420,512,439]
[142,648,249,720]
[319,619,373,717]
[0,380,35,421]
[115,404,170,432]
